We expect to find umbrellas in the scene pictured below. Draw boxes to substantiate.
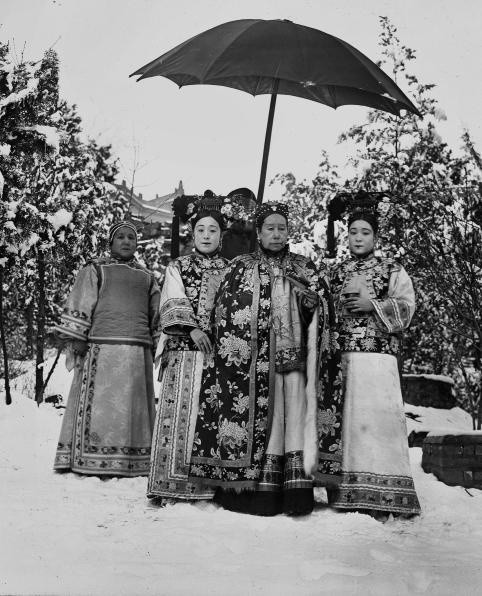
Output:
[130,19,423,259]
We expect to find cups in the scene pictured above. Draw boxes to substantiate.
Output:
[341,292,361,313]
